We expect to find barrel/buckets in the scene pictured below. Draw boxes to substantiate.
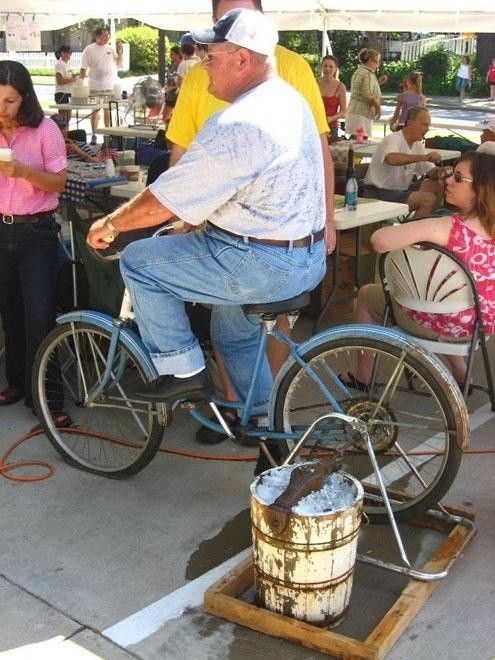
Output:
[71,86,90,99]
[250,454,369,631]
[114,149,136,166]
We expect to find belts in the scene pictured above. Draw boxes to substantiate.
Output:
[0,211,53,225]
[206,219,327,248]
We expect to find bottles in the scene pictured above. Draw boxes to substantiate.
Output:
[105,158,116,176]
[122,90,127,100]
[345,173,359,210]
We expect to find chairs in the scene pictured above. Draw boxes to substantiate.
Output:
[368,241,495,415]
[55,130,171,316]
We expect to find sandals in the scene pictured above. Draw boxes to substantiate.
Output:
[31,408,71,428]
[0,388,26,406]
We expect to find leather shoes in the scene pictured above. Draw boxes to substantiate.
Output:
[131,367,216,403]
[253,434,293,475]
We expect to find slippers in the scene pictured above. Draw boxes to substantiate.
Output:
[338,370,374,393]
[455,382,475,397]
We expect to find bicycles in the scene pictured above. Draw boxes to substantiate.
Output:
[31,218,470,528]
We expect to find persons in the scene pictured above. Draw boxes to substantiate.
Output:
[316,55,346,137]
[456,57,471,102]
[50,29,208,163]
[0,60,72,427]
[344,49,388,137]
[390,71,426,132]
[164,1,338,448]
[86,7,327,476]
[362,107,449,220]
[486,59,495,100]
[338,151,495,396]
[475,120,495,155]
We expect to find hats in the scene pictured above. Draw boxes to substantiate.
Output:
[190,7,279,58]
[181,33,196,46]
[51,114,69,129]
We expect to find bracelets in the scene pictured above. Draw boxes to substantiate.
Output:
[106,214,115,231]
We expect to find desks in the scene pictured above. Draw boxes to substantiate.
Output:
[49,98,134,130]
[94,119,375,191]
[107,171,408,335]
[353,142,462,195]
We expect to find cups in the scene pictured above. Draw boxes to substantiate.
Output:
[113,84,121,100]
[117,150,140,181]
[355,128,364,144]
[70,87,89,97]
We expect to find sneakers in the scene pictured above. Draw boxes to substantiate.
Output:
[196,404,273,447]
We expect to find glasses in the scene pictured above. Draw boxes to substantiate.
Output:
[450,172,475,183]
